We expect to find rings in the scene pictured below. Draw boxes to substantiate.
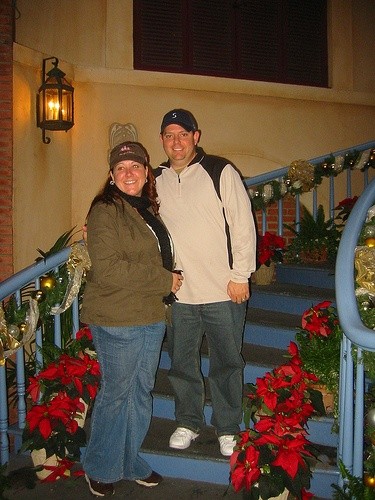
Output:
[242,298,245,301]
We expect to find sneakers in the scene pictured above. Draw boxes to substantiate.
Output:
[135,470,161,488]
[85,472,115,497]
[168,427,200,449]
[217,435,237,457]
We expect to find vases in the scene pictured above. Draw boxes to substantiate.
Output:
[254,262,275,284]
[254,482,290,500]
[51,396,89,429]
[306,385,336,412]
[30,448,69,480]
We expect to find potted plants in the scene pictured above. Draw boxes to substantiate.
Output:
[284,205,332,262]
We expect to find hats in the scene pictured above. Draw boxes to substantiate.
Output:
[161,110,196,134]
[110,144,148,170]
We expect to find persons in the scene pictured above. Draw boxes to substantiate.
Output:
[82,109,257,456]
[78,141,183,498]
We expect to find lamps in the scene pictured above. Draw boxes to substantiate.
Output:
[36,56,75,144]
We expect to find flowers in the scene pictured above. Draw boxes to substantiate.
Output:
[24,190,364,500]
